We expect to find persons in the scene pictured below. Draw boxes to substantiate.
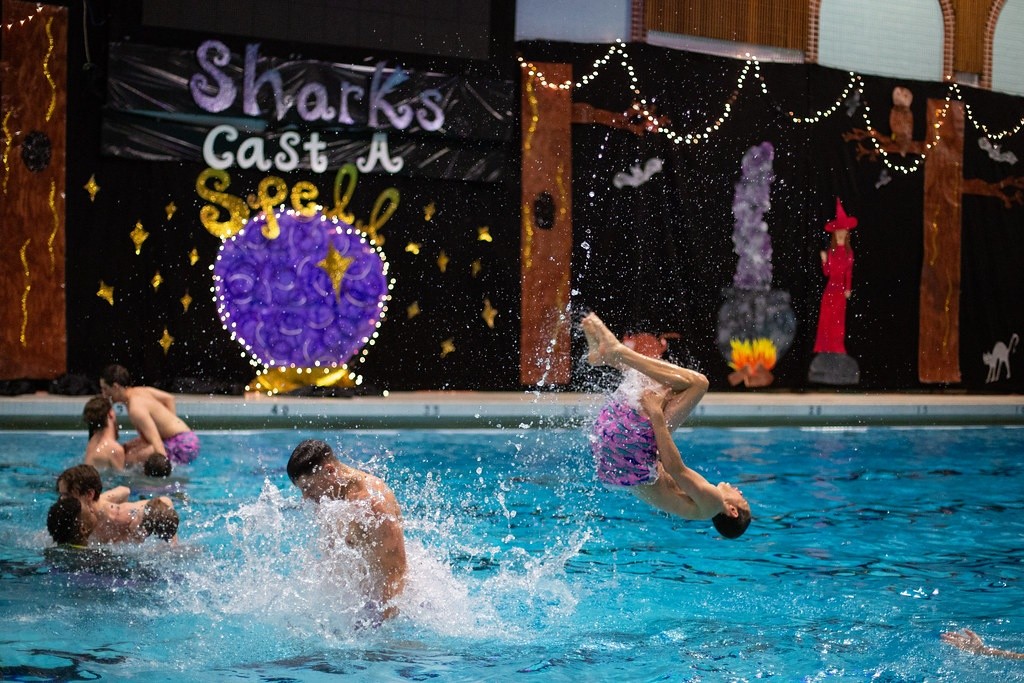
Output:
[56,460,172,561]
[287,438,409,636]
[119,441,181,484]
[99,362,201,472]
[82,395,128,481]
[45,494,200,567]
[579,311,754,539]
